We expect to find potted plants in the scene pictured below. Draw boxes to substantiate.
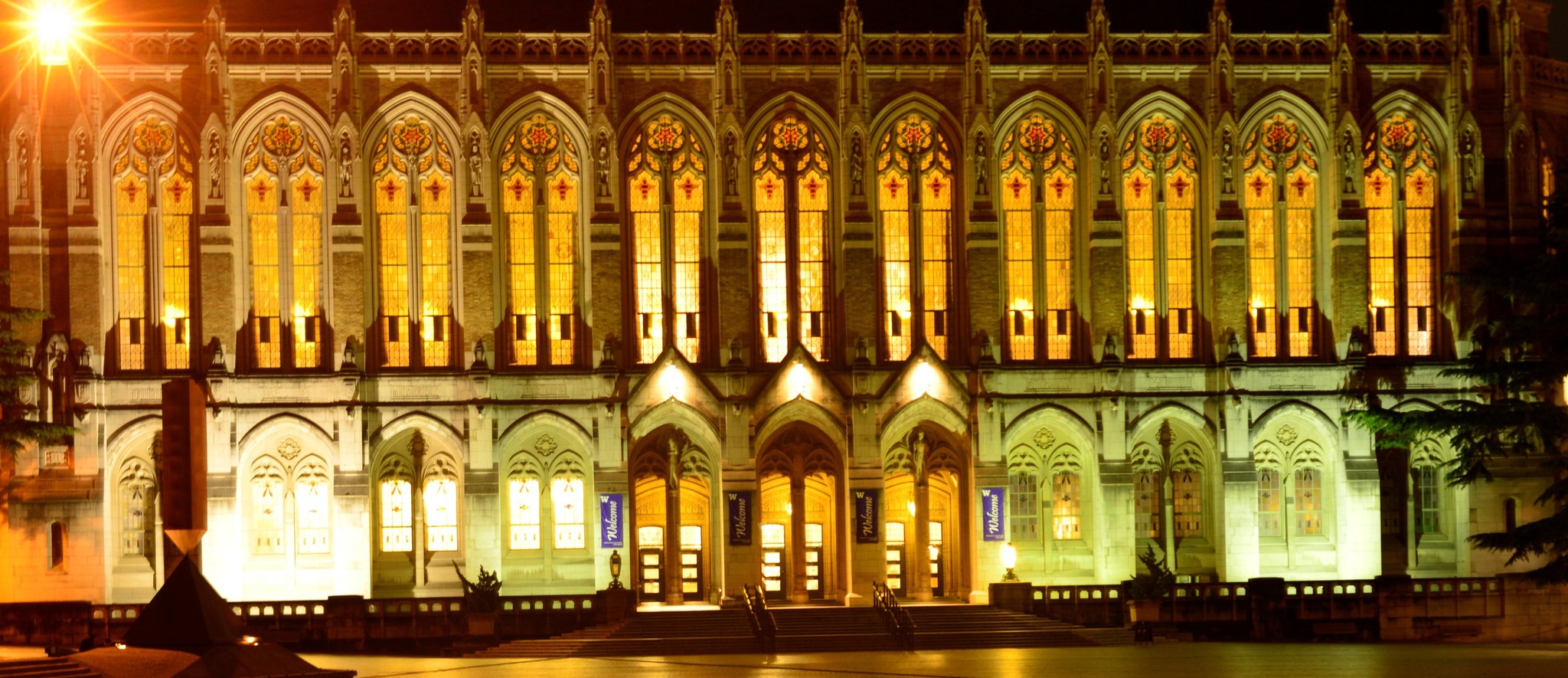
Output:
[1127,542,1175,622]
[452,559,503,634]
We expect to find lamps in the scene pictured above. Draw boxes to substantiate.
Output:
[607,549,625,589]
